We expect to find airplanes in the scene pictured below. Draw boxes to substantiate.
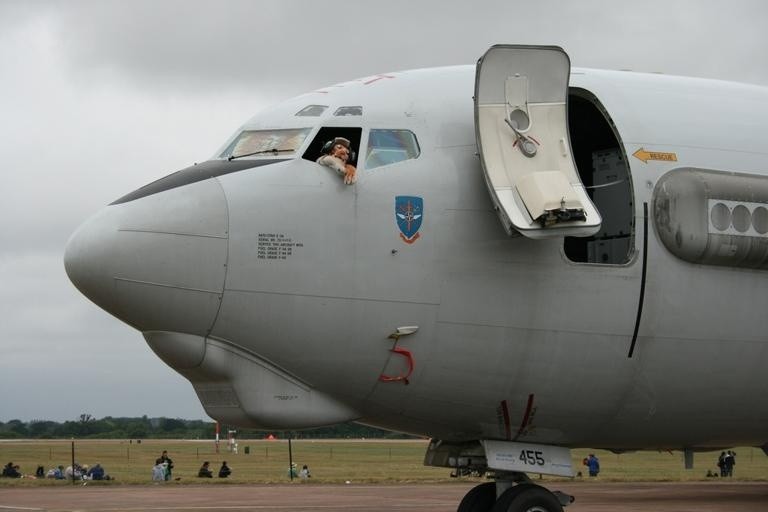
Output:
[62,43,768,512]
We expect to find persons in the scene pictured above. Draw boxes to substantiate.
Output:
[717,450,736,477]
[316,137,357,184]
[288,462,311,479]
[219,461,232,477]
[198,461,212,478]
[2,462,105,480]
[152,450,174,481]
[583,453,600,476]
